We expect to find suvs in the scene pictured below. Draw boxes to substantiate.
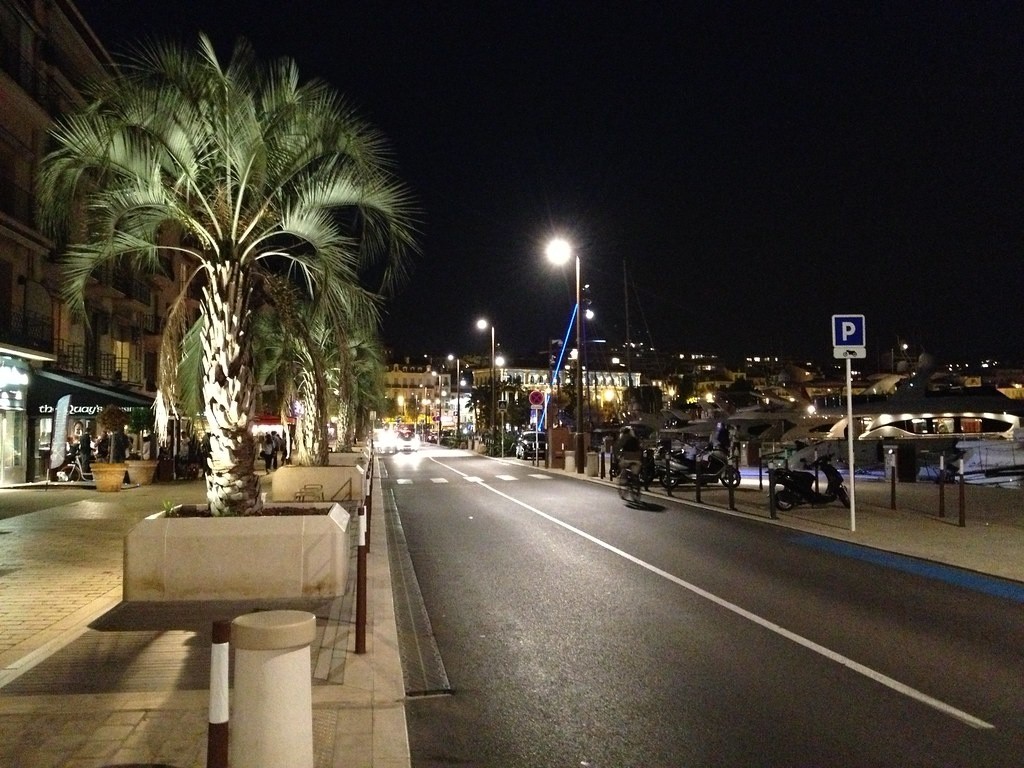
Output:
[516,431,545,460]
[426,432,437,444]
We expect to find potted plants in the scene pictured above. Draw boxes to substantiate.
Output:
[123,406,160,485]
[180,267,385,501]
[294,305,374,466]
[89,404,131,493]
[29,36,426,600]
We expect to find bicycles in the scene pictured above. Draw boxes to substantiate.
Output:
[613,451,641,504]
[61,453,103,482]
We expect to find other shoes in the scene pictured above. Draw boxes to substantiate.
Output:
[266,468,270,475]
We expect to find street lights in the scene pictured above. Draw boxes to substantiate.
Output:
[431,370,447,446]
[411,384,431,442]
[478,319,505,456]
[546,238,595,474]
[448,354,467,438]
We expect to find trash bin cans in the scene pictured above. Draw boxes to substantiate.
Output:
[603,436,613,453]
[565,451,576,472]
[586,452,599,477]
[232,609,317,767]
[883,444,916,482]
[158,455,174,482]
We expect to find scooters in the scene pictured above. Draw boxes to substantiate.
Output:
[765,452,850,512]
[654,423,742,489]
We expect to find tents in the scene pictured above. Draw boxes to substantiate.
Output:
[255,412,297,460]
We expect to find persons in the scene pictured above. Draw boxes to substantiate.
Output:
[639,450,657,492]
[66,424,293,481]
[717,422,745,457]
[612,428,642,461]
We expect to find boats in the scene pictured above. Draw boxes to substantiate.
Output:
[659,411,1024,485]
